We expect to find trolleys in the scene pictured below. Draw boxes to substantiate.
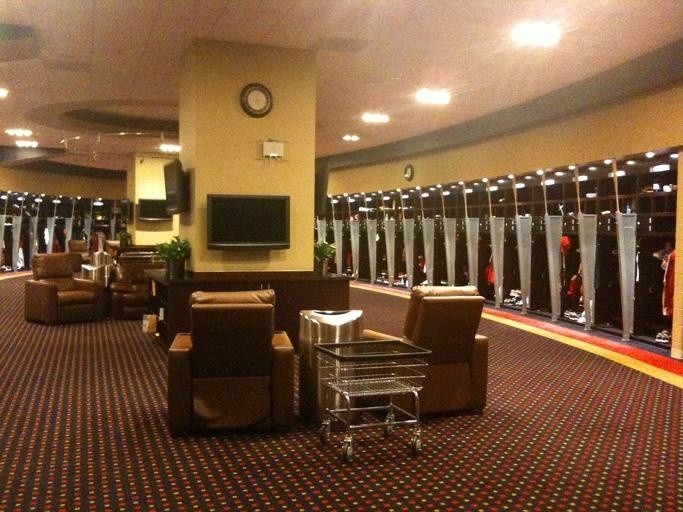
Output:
[313,338,432,463]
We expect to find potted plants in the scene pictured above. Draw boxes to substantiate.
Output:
[115,232,132,249]
[152,235,191,281]
[314,239,337,274]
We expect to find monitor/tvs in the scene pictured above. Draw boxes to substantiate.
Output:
[163,158,190,215]
[205,194,293,249]
[139,198,173,223]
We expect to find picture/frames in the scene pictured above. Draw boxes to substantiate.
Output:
[403,165,414,181]
[239,82,273,118]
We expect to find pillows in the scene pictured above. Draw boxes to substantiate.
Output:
[187,289,277,336]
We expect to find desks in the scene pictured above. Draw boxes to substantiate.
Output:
[143,267,350,355]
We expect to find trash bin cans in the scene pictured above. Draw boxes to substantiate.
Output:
[80,251,107,288]
[298,310,363,424]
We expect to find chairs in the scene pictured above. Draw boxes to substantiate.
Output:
[109,258,164,320]
[24,252,107,326]
[53,252,82,272]
[164,288,298,438]
[104,239,121,257]
[67,238,93,258]
[361,283,489,420]
